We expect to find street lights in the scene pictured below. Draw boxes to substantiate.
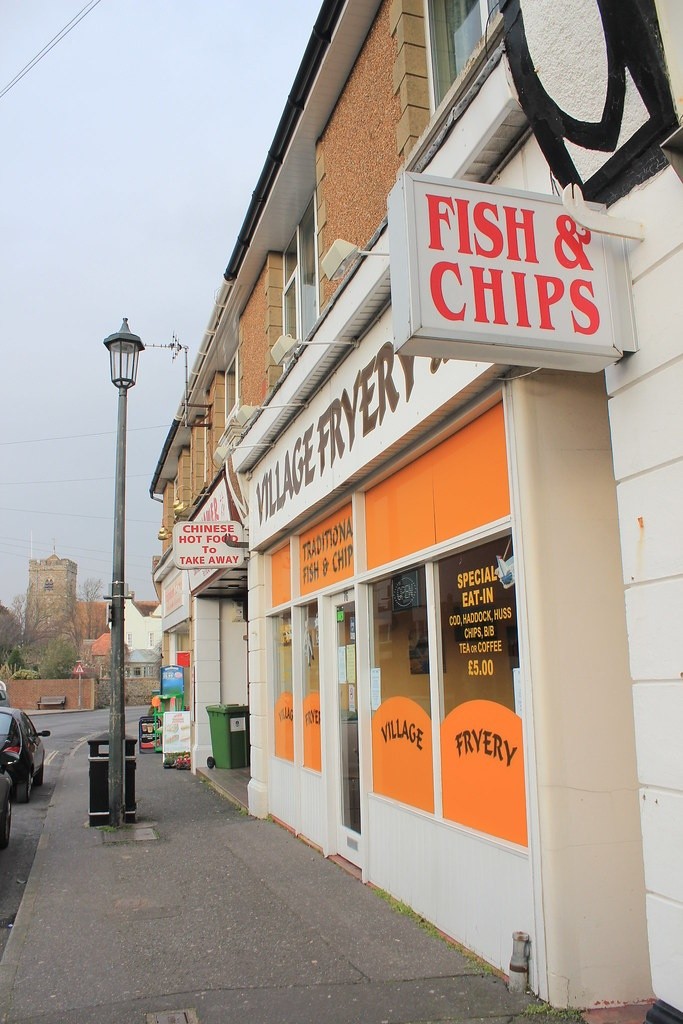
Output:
[102,316,147,826]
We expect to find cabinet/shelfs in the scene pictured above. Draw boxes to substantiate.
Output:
[154,694,185,751]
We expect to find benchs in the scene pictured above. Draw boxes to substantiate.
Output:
[36,696,66,710]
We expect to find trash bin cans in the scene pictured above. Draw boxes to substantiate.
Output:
[87,739,138,827]
[205,704,249,769]
[152,690,160,697]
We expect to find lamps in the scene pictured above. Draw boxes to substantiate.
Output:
[173,485,211,515]
[158,513,177,541]
[270,334,360,365]
[212,443,274,469]
[322,238,390,282]
[237,403,309,428]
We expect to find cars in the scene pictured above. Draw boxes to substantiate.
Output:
[0,739,21,848]
[0,707,51,803]
